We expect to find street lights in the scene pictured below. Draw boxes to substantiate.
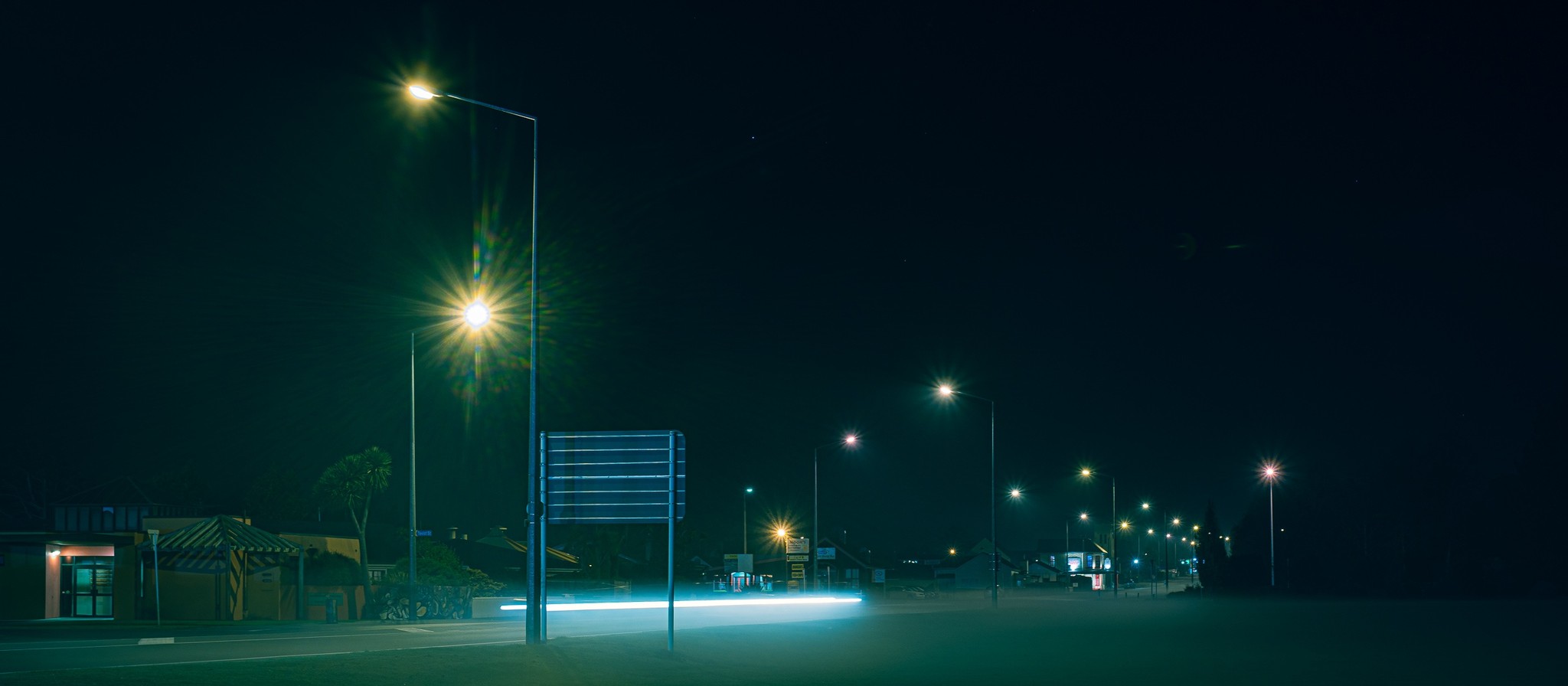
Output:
[1108,521,1131,573]
[1173,518,1194,584]
[406,299,494,621]
[812,434,858,591]
[1174,536,1187,569]
[1135,527,1155,583]
[1143,502,1170,592]
[1265,463,1275,589]
[1081,469,1118,597]
[743,487,754,555]
[940,384,999,608]
[403,81,543,652]
[1157,533,1172,574]
[1064,513,1088,578]
